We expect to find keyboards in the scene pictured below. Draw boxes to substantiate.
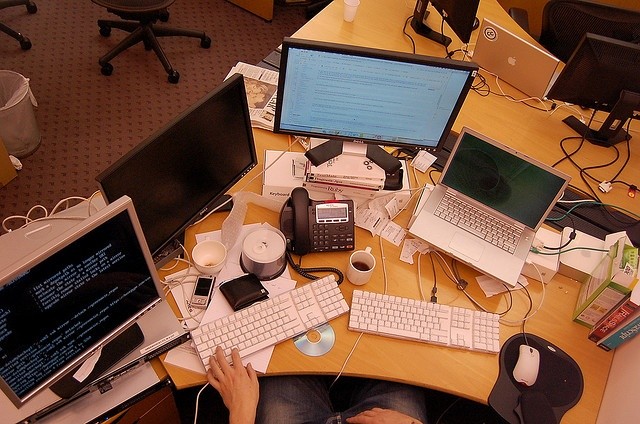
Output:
[347,289,502,356]
[188,273,350,373]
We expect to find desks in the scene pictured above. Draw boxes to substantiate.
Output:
[133,61,619,424]
[265,0,639,240]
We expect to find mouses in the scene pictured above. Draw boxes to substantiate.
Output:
[513,342,543,389]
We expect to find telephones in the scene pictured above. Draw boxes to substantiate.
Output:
[278,186,355,286]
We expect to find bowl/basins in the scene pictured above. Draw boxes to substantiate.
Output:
[192,240,227,274]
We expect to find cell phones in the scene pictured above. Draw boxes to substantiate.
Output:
[189,275,216,309]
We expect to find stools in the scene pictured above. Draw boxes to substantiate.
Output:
[94,0,211,82]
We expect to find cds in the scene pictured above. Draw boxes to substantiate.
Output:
[292,322,336,356]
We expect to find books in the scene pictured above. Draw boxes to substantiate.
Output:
[589,280,640,352]
[302,137,386,198]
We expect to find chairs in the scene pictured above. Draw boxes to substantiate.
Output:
[511,1,640,78]
[1,1,36,52]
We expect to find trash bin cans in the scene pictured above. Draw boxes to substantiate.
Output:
[0,69,42,159]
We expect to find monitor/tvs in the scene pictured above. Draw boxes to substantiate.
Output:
[546,30,637,146]
[273,34,477,172]
[410,1,480,45]
[1,194,167,410]
[68,73,259,257]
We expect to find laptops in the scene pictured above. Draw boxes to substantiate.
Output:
[470,17,560,101]
[402,126,577,287]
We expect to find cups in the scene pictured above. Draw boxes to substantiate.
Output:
[347,247,376,286]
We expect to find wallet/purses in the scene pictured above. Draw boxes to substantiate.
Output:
[219,273,269,312]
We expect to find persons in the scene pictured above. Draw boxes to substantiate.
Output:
[205,345,431,423]
